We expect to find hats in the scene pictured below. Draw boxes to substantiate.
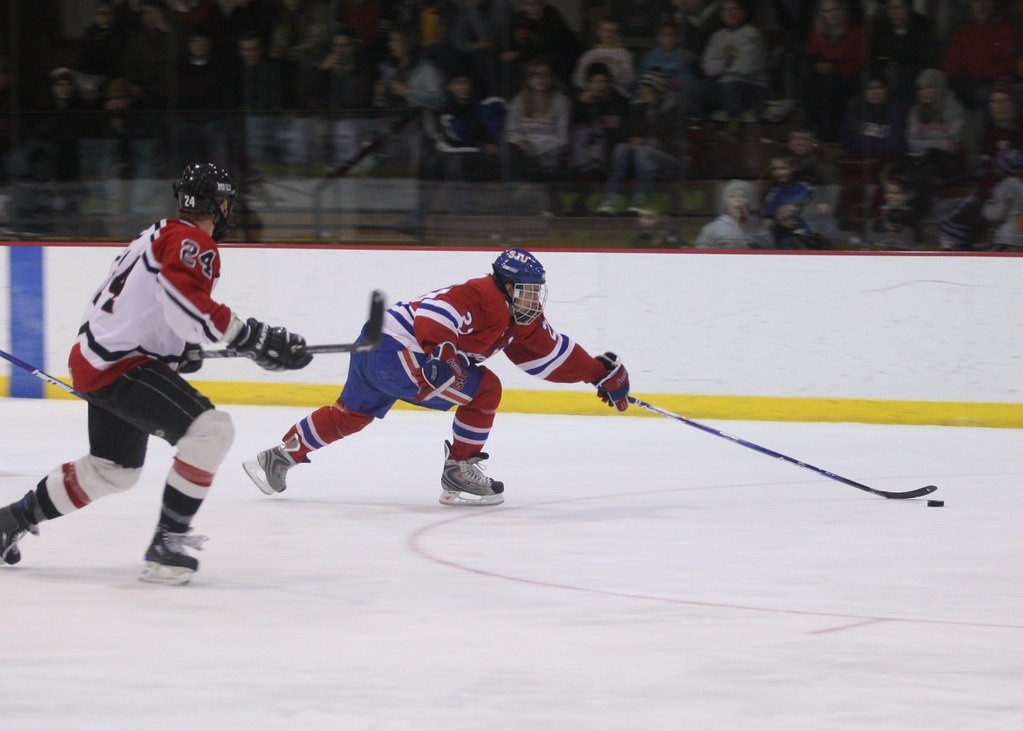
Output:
[587,62,609,75]
[722,180,753,203]
[637,65,668,94]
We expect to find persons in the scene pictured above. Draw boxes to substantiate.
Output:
[0,161,315,585]
[240,248,629,506]
[28,0,1023,254]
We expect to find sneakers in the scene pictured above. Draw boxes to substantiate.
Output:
[242,433,301,496]
[140,528,209,585]
[0,491,39,565]
[440,440,505,505]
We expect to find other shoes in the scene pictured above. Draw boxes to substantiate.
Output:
[596,204,619,214]
[628,205,657,218]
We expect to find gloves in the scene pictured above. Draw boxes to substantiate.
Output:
[227,318,312,371]
[593,352,630,412]
[423,341,470,392]
[177,340,203,373]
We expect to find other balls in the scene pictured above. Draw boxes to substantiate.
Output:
[928,500,944,507]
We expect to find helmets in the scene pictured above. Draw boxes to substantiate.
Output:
[177,162,237,239]
[494,249,549,325]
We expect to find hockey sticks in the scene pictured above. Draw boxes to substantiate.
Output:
[184,289,385,362]
[0,349,177,445]
[598,385,938,500]
